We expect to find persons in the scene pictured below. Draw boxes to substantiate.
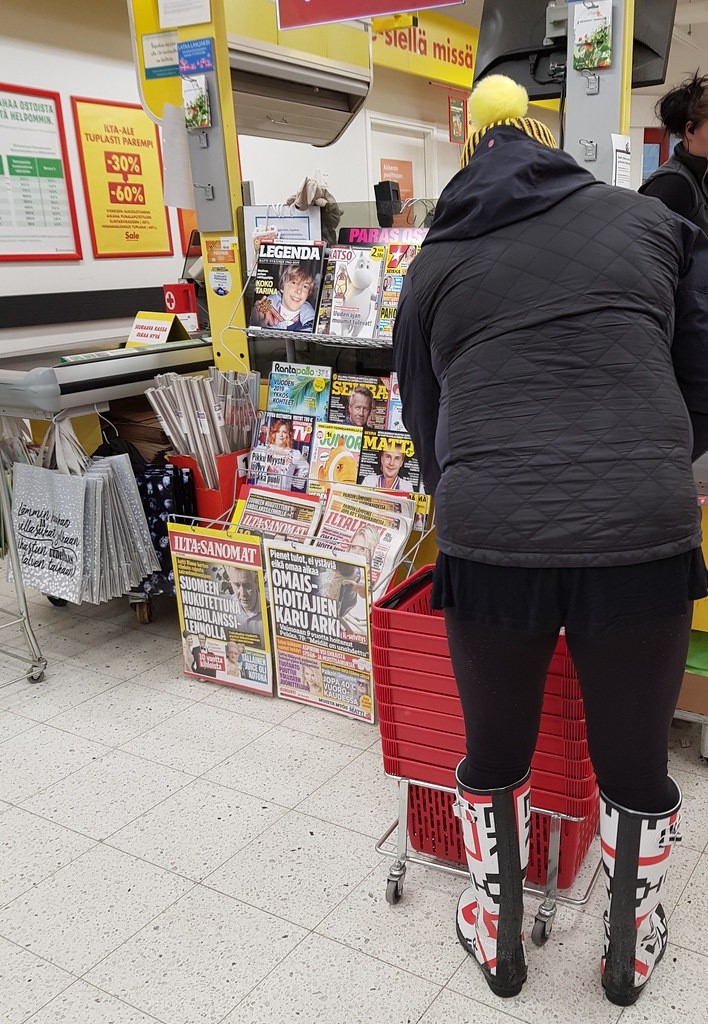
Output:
[626,66,708,250]
[176,514,388,700]
[389,78,708,1007]
[244,260,415,498]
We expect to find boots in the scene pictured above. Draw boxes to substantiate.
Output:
[599,773,683,1006]
[454,755,531,998]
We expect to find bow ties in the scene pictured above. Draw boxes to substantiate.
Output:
[201,647,206,651]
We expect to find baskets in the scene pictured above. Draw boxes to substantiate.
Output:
[371,564,598,888]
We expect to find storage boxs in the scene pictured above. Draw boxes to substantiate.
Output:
[163,448,250,530]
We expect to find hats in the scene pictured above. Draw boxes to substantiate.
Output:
[460,73,559,169]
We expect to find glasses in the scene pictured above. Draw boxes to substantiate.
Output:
[356,681,368,687]
[686,74,708,117]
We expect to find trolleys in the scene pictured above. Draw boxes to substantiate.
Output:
[370,768,604,946]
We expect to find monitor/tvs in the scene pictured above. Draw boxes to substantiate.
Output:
[472,0,677,99]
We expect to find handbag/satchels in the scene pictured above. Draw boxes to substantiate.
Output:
[1,415,199,607]
